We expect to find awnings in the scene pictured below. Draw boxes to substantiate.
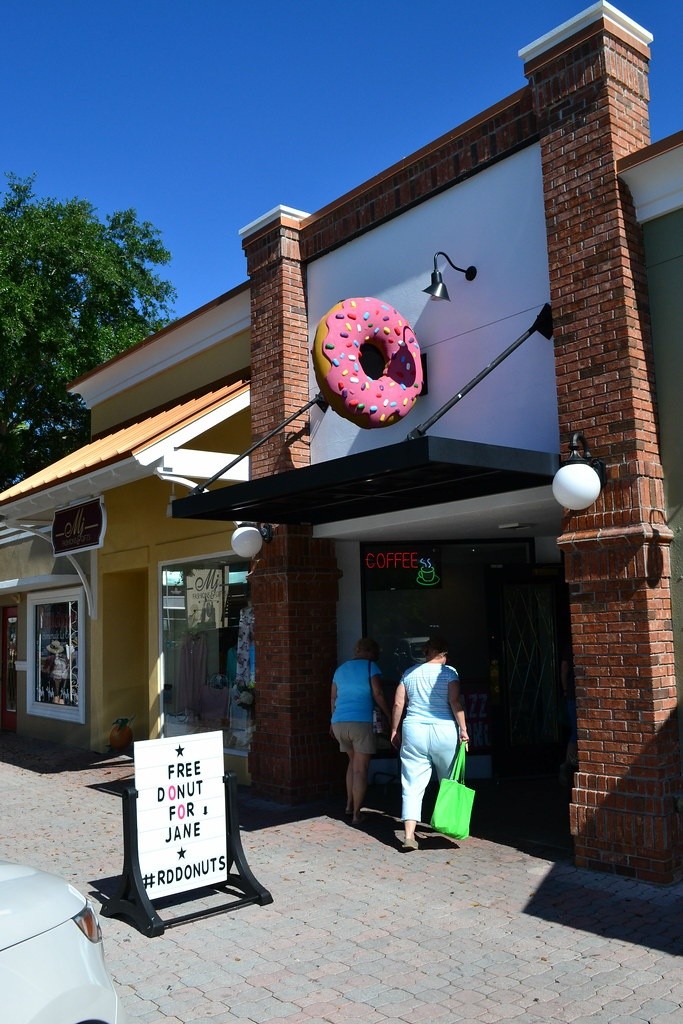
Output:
[171,319,557,524]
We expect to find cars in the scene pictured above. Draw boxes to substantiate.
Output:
[1,863,124,1024]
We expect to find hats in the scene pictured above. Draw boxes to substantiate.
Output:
[46,640,65,655]
[71,637,78,646]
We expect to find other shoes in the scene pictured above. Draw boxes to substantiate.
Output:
[401,839,419,851]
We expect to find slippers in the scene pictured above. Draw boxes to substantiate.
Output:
[352,816,367,825]
[344,809,353,815]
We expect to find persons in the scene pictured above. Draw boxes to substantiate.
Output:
[330,638,390,823]
[390,640,470,849]
[560,642,577,786]
[43,638,77,705]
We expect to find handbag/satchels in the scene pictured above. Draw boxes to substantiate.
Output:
[373,707,392,738]
[429,739,477,842]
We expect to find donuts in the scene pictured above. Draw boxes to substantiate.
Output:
[312,296,422,428]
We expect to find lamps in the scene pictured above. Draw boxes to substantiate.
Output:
[165,482,177,518]
[553,431,602,510]
[421,251,477,302]
[231,521,273,557]
